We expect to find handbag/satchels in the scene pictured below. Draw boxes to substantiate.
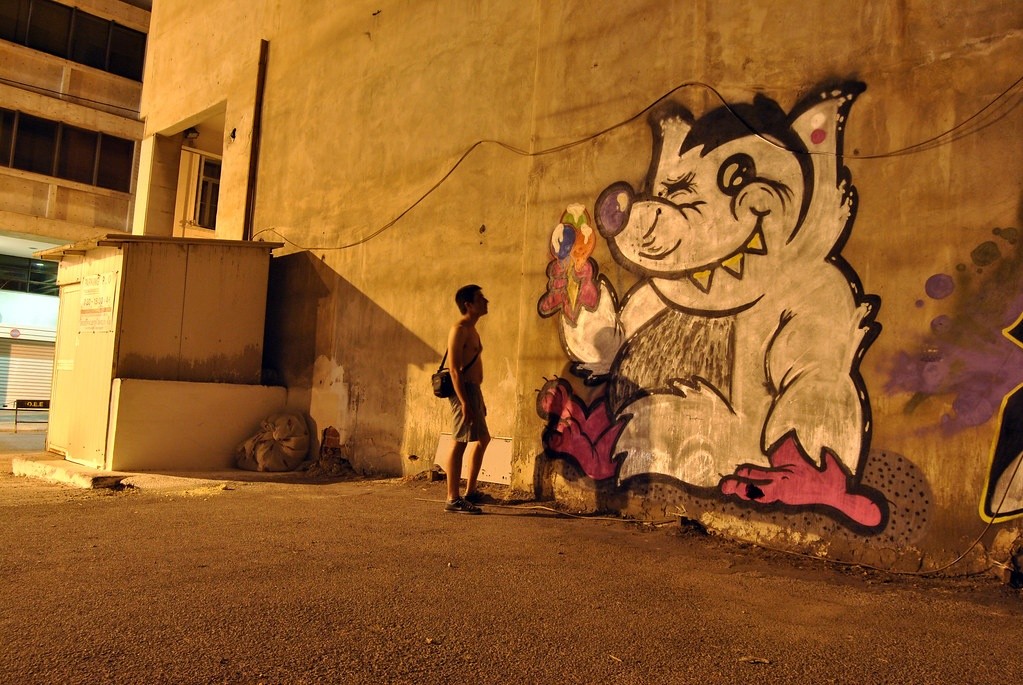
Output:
[431,371,454,398]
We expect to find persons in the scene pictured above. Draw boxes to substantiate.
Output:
[444,284,497,514]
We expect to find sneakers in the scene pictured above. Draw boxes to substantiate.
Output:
[462,490,497,504]
[443,495,482,514]
